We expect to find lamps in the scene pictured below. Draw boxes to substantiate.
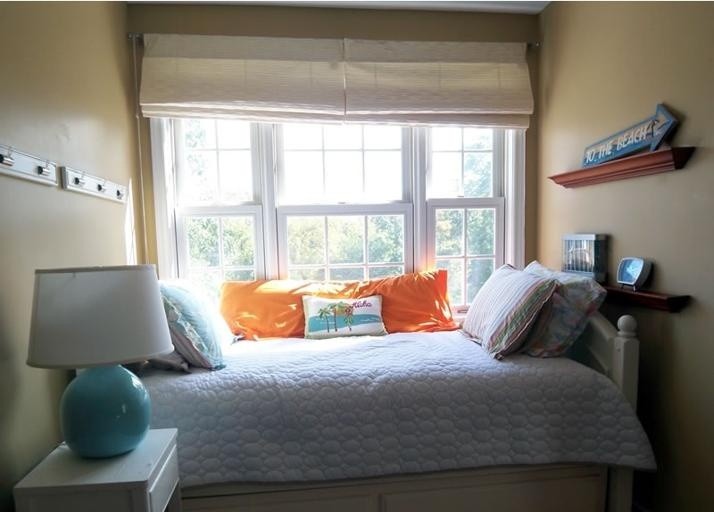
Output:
[27,264,175,458]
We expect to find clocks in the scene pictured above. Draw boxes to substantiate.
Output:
[616,257,651,291]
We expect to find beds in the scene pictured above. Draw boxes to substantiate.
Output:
[64,304,640,512]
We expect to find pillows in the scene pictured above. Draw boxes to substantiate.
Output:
[160,281,223,370]
[302,295,389,339]
[524,260,606,358]
[462,265,556,359]
[161,350,189,372]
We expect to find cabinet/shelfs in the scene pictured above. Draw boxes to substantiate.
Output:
[547,146,695,313]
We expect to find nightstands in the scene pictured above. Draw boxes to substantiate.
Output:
[12,428,181,512]
